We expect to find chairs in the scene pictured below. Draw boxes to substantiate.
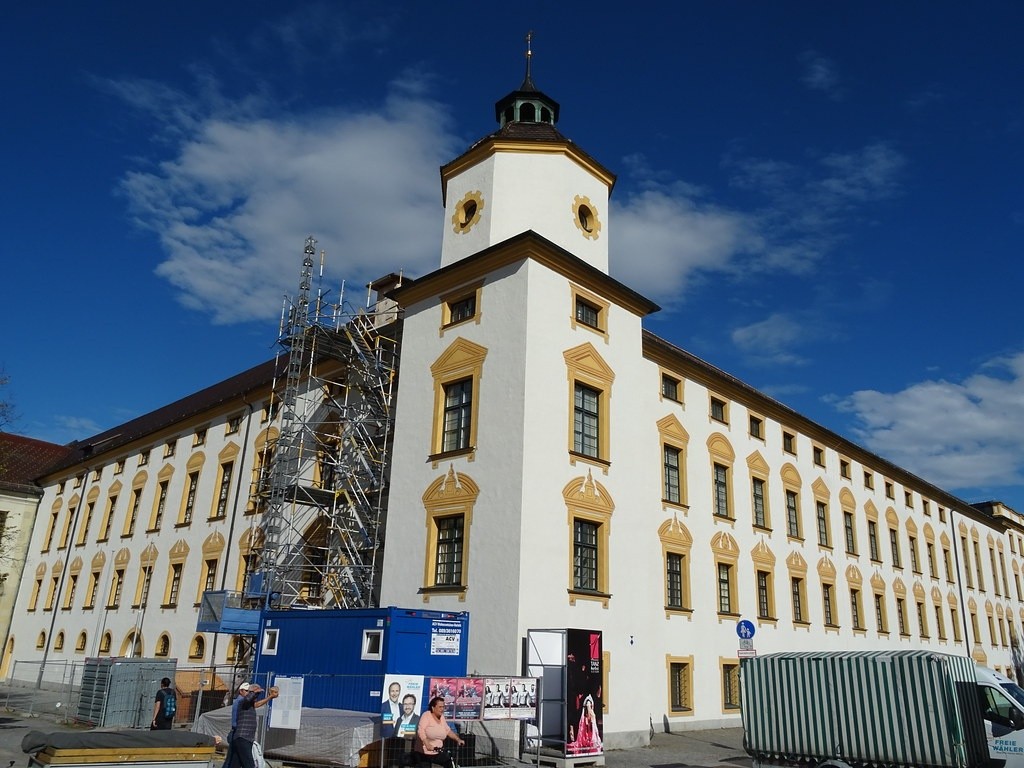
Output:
[983,693,1002,721]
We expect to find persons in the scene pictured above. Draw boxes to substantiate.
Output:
[223,683,250,768]
[414,698,465,768]
[484,684,535,708]
[381,682,404,736]
[150,678,177,731]
[228,684,278,768]
[394,694,420,738]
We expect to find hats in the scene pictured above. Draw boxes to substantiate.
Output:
[249,684,264,692]
[239,682,250,690]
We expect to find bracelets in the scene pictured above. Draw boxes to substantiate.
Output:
[152,720,155,721]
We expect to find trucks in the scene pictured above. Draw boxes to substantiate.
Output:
[741,650,1024,768]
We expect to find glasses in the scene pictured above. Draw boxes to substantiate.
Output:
[403,703,414,706]
[244,690,248,691]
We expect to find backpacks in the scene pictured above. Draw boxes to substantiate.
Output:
[160,689,177,717]
